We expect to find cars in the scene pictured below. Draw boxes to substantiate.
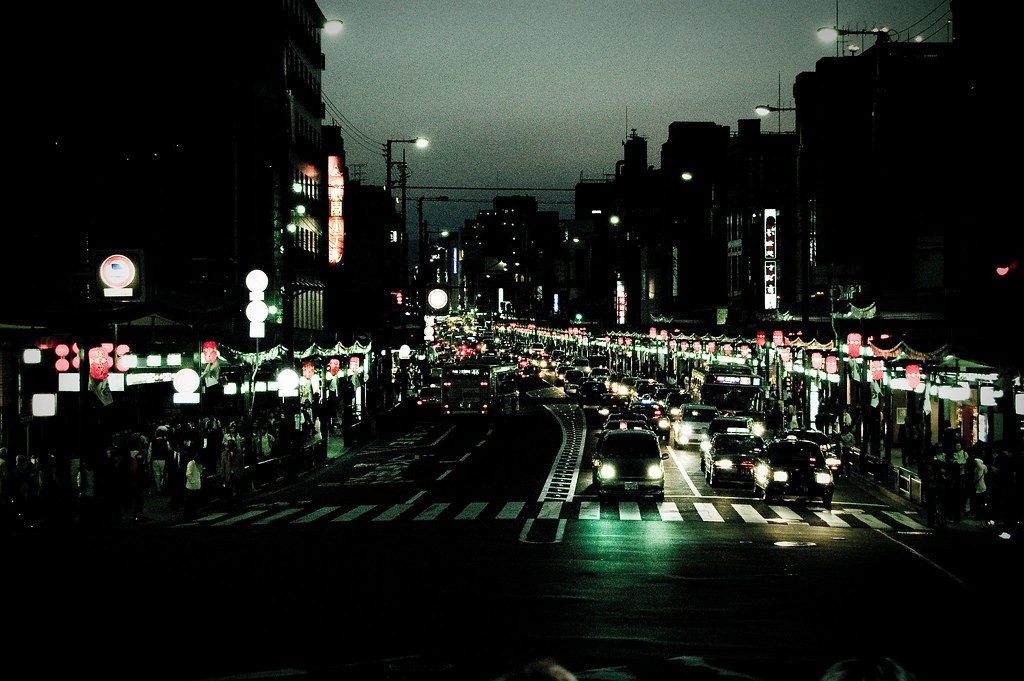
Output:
[749,438,836,505]
[777,428,842,479]
[698,431,768,489]
[416,316,767,456]
[591,430,669,504]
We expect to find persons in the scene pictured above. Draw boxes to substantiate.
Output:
[819,387,894,462]
[0,400,314,524]
[796,388,802,430]
[897,416,1024,529]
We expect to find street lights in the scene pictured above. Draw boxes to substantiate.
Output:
[384,135,431,433]
[753,103,811,431]
